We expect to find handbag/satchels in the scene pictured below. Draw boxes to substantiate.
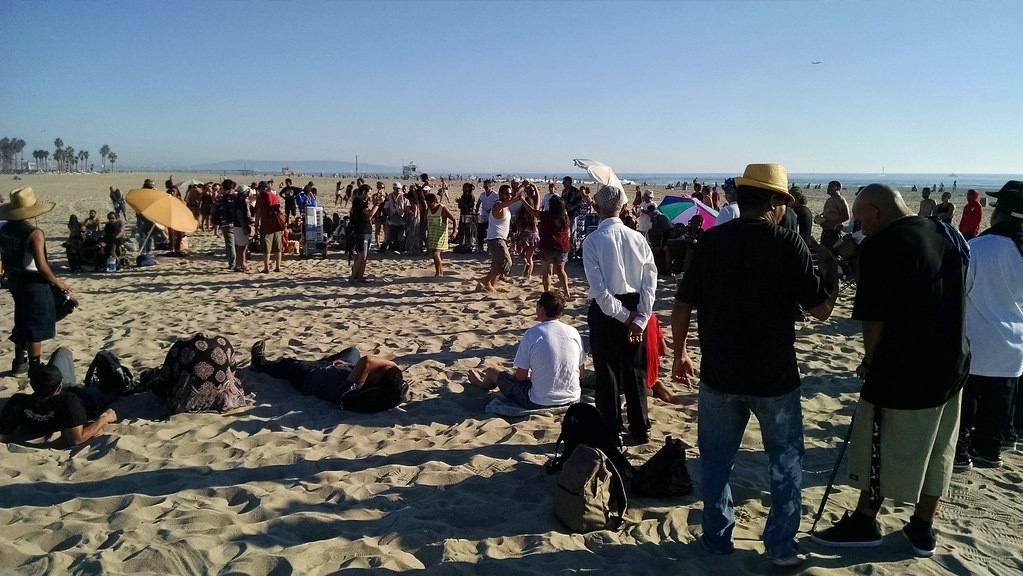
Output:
[261,211,286,234]
[50,282,79,322]
[633,437,693,496]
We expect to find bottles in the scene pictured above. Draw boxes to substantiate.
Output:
[132,373,137,389]
[123,373,130,390]
[282,240,299,254]
[107,256,116,272]
[641,205,643,214]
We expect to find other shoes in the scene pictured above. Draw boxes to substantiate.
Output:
[357,278,369,283]
[349,275,355,285]
[523,263,530,277]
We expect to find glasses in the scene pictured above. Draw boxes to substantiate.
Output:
[503,192,514,196]
[378,187,385,189]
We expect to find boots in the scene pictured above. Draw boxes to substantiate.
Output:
[954,428,973,470]
[970,433,1004,466]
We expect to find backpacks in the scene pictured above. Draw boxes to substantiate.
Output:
[547,404,628,476]
[84,350,136,396]
[555,444,627,532]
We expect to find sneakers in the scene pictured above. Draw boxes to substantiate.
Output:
[811,509,883,546]
[902,515,936,556]
[251,340,265,371]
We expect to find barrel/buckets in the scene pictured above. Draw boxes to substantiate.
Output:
[980,198,986,207]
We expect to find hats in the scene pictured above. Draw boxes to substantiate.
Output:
[985,180,1023,198]
[237,185,247,193]
[594,186,623,211]
[0,186,56,220]
[393,182,402,188]
[646,189,654,198]
[735,164,795,207]
[563,176,572,184]
[423,186,431,190]
[985,190,1023,218]
[255,181,268,189]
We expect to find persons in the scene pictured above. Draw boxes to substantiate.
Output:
[61,210,125,274]
[0,185,79,381]
[959,189,983,242]
[0,346,139,446]
[455,175,958,298]
[937,191,956,225]
[331,173,457,286]
[953,180,1023,473]
[810,183,973,557]
[467,291,587,411]
[583,186,659,448]
[110,186,127,222]
[251,340,409,415]
[136,174,187,252]
[671,164,838,571]
[778,186,812,241]
[145,333,246,413]
[814,181,849,250]
[834,186,871,234]
[184,173,333,274]
[918,188,939,218]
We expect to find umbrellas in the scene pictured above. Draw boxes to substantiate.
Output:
[125,188,199,255]
[574,158,628,204]
[657,194,720,232]
